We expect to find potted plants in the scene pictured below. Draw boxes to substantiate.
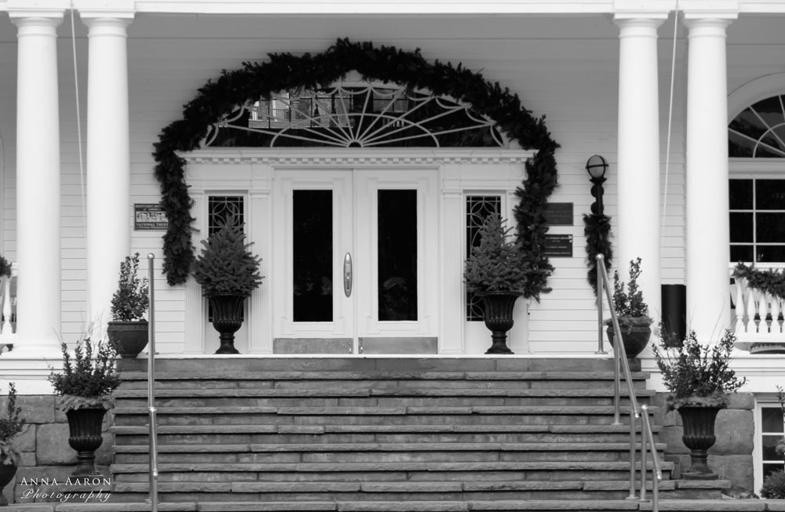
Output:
[48,250,147,482]
[192,213,268,356]
[1,381,26,506]
[464,210,532,353]
[606,253,751,482]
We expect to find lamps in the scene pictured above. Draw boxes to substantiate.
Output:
[586,151,606,217]
